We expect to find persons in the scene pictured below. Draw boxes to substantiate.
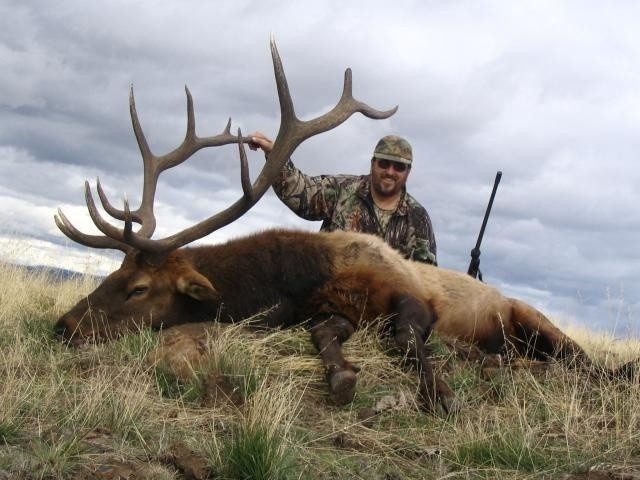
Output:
[243,129,438,268]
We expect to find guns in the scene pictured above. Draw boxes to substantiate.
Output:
[468,171,502,279]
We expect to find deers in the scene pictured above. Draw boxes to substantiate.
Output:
[54,30,639,418]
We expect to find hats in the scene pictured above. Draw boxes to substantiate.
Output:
[372,134,413,165]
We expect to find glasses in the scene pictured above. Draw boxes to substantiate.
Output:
[375,158,409,173]
